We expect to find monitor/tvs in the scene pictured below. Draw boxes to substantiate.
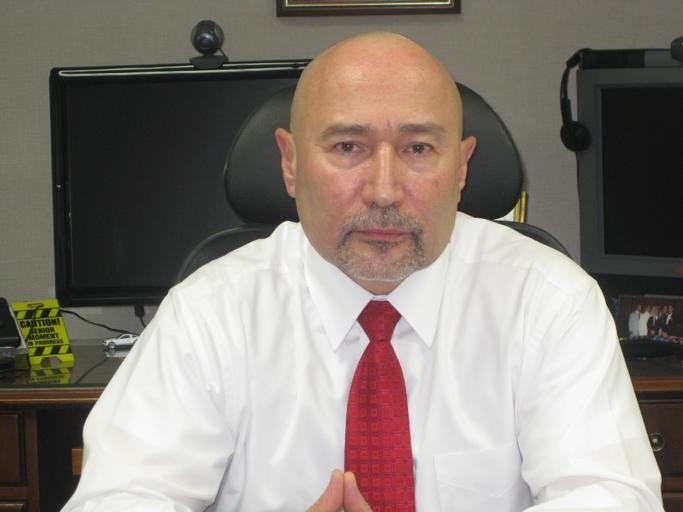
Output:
[48,61,313,308]
[574,64,683,283]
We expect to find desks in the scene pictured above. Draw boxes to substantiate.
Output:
[0,338,683,512]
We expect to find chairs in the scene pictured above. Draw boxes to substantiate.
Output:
[172,83,572,288]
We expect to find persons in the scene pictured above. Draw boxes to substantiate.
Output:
[627,304,677,338]
[60,28,661,512]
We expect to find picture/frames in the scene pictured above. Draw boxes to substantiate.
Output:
[495,190,526,223]
[275,0,461,16]
[615,293,683,349]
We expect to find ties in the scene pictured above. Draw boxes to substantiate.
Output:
[342,299,417,512]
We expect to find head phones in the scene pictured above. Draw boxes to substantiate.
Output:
[557,48,592,152]
